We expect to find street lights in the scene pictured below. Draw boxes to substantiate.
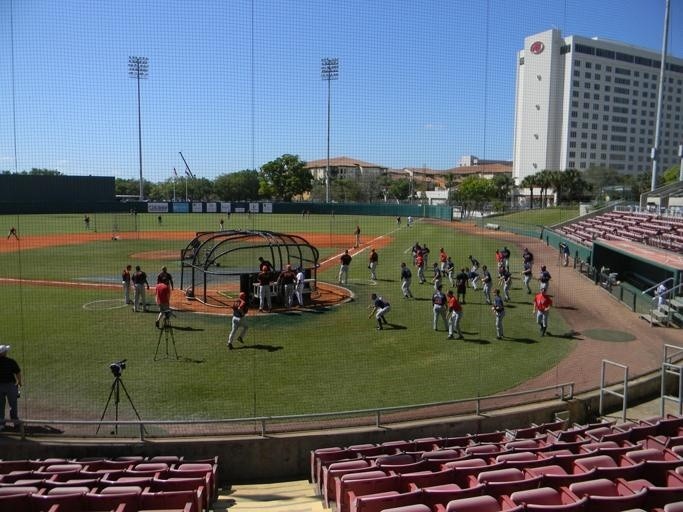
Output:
[127,50,149,203]
[320,54,339,205]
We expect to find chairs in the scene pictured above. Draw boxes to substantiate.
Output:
[1,453,226,512]
[558,199,682,254]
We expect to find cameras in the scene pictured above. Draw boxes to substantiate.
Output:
[109,359,127,377]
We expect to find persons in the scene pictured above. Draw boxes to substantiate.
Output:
[365,294,392,330]
[560,242,570,266]
[353,224,361,247]
[338,249,352,284]
[219,218,224,230]
[257,266,273,313]
[369,248,378,280]
[292,267,305,307]
[84,215,90,229]
[155,277,170,328]
[227,292,249,349]
[131,266,149,312]
[0,343,22,429]
[397,215,401,224]
[399,241,551,340]
[157,265,174,290]
[259,257,274,272]
[276,265,297,308]
[6,224,20,241]
[157,214,162,225]
[407,215,414,228]
[122,265,133,304]
[657,281,669,309]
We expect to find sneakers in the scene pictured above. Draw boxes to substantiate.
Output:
[237,336,243,343]
[227,343,233,350]
[155,320,159,328]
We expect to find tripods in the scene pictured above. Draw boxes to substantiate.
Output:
[153,317,179,360]
[94,377,148,436]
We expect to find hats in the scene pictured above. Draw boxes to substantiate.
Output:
[0,345,10,353]
